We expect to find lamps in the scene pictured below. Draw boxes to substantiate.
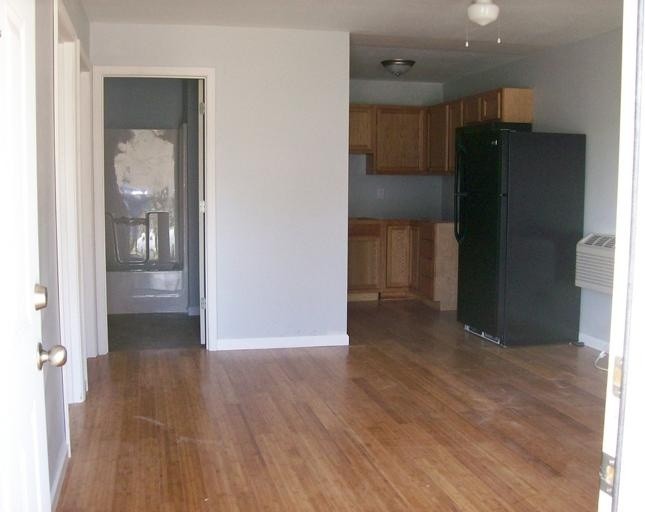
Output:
[382,59,415,78]
[467,0,499,28]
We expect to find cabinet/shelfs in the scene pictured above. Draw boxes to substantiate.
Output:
[410,223,421,298]
[365,106,427,174]
[348,221,384,300]
[428,100,462,176]
[386,224,409,294]
[462,89,534,125]
[349,104,376,155]
[418,224,458,310]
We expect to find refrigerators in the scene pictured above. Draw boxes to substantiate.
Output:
[455,128,587,349]
